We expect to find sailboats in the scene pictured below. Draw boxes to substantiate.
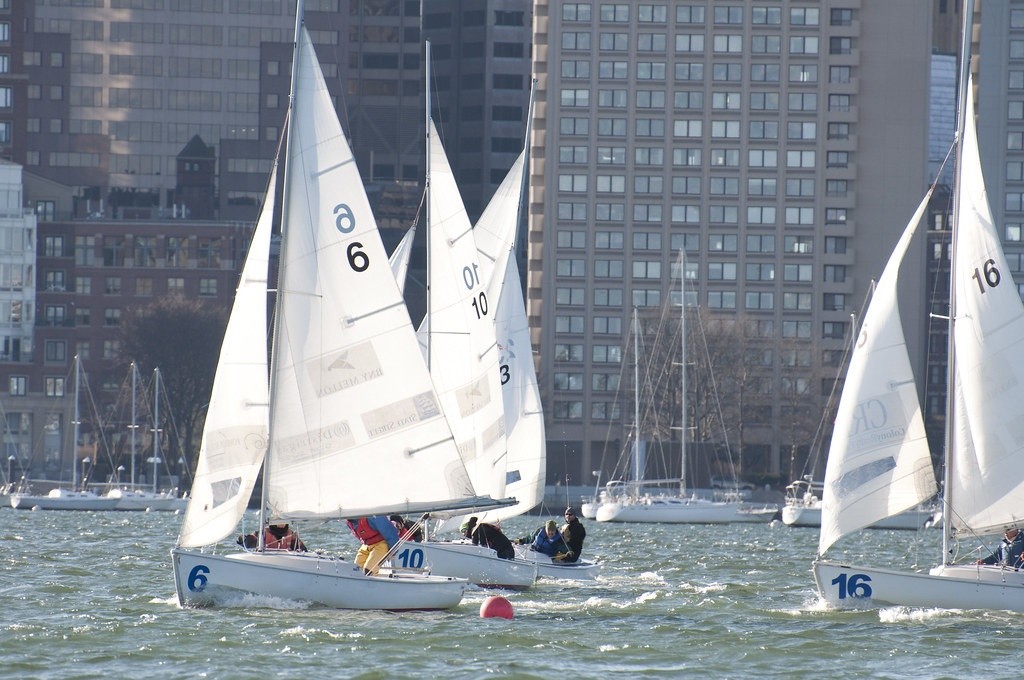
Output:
[813,72,1023,614]
[100,366,193,512]
[1,352,165,512]
[581,302,714,518]
[597,244,776,525]
[782,277,935,531]
[167,0,597,614]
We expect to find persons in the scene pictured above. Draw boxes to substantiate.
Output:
[977,523,1024,571]
[514,520,574,561]
[558,507,586,561]
[391,515,422,543]
[460,517,514,559]
[237,523,308,552]
[346,515,399,576]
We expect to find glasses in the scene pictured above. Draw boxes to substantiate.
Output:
[566,513,573,516]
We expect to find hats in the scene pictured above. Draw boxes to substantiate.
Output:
[546,520,556,528]
[460,522,469,532]
[566,507,575,516]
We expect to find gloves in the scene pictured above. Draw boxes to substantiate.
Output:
[565,551,574,558]
